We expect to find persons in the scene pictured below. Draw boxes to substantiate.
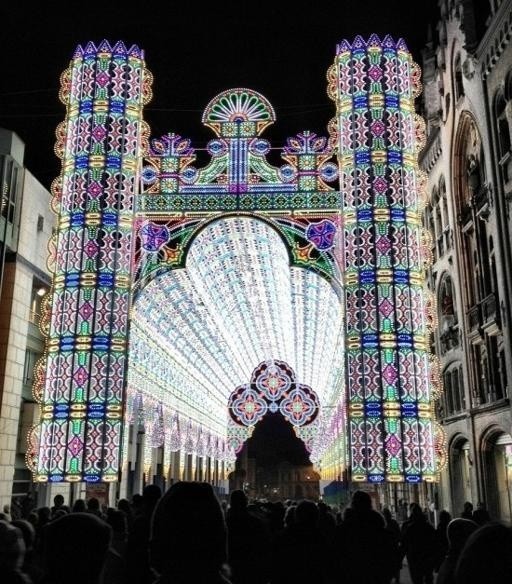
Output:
[0,475,512,584]
